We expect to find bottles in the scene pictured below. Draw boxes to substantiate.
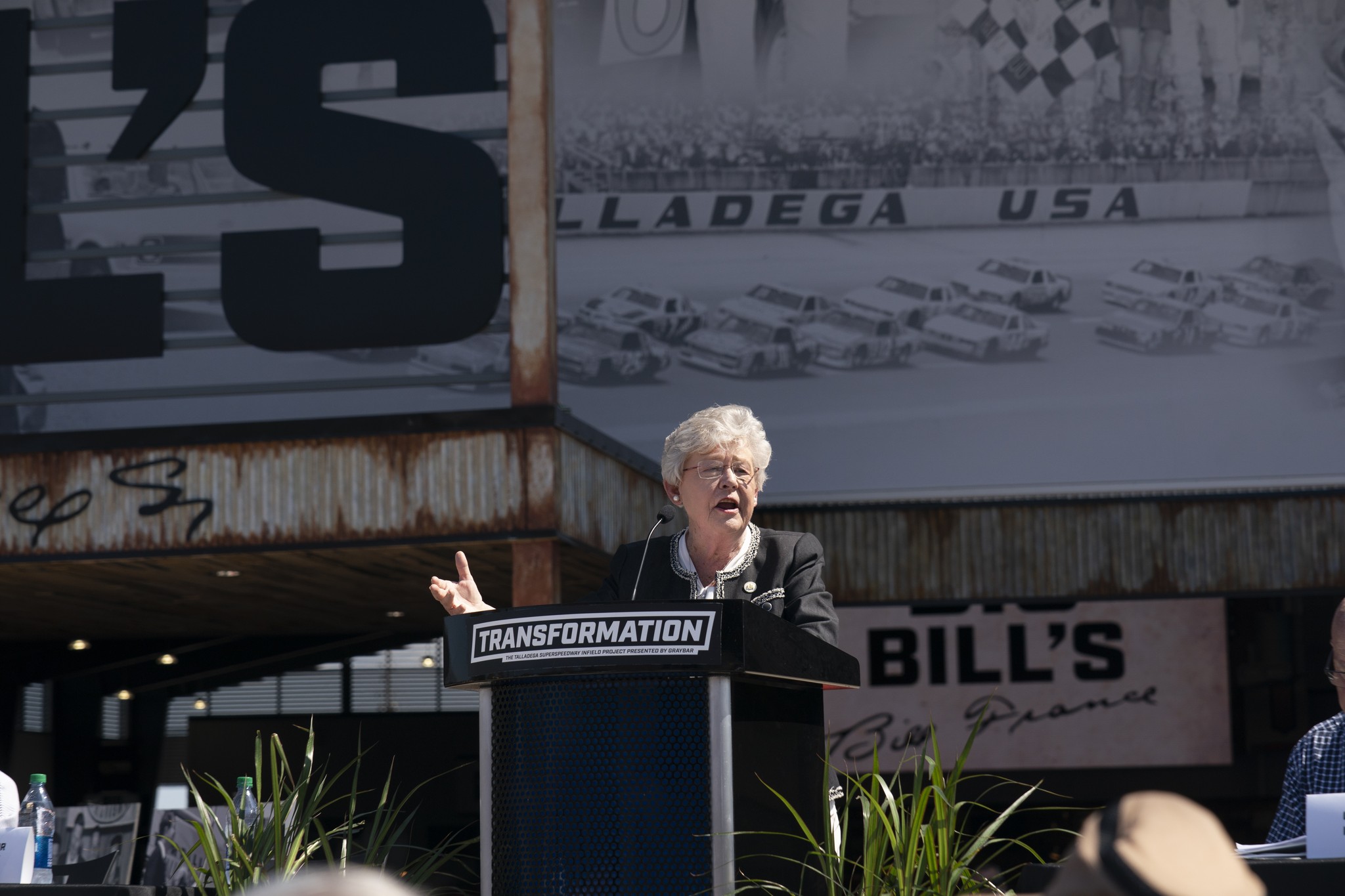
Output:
[225,777,260,889]
[18,774,56,884]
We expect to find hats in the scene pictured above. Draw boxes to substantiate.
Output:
[1042,791,1267,895]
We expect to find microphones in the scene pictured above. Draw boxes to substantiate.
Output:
[632,505,676,602]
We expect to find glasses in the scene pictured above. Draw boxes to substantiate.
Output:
[1326,653,1345,688]
[684,458,760,478]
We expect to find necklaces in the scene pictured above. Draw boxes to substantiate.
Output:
[686,532,745,598]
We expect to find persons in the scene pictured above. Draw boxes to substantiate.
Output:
[144,812,176,896]
[52,813,123,886]
[239,862,414,896]
[1267,596,1345,843]
[1045,792,1266,896]
[430,405,839,649]
